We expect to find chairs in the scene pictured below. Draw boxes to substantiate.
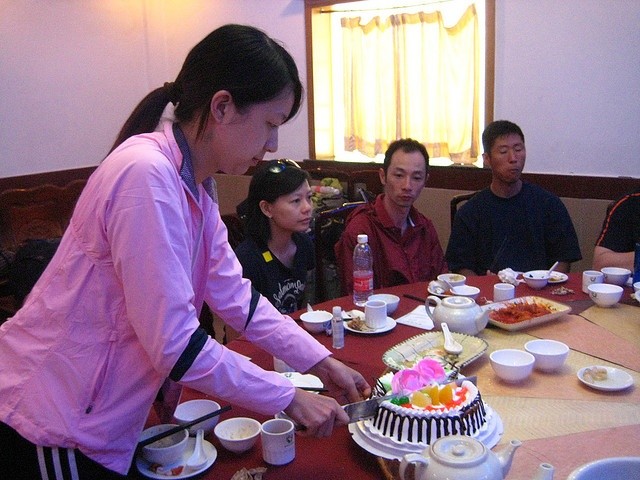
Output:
[449,191,476,231]
[0,236,60,324]
[220,213,246,250]
[313,201,363,303]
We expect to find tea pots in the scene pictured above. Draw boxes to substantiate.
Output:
[398,434,522,480]
[425,295,496,336]
[534,462,555,480]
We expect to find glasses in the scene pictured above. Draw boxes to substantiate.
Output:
[268,159,302,173]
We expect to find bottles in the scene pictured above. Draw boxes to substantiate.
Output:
[331,305,346,350]
[310,185,340,197]
[353,233,375,307]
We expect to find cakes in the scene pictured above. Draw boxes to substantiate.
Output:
[363,356,494,447]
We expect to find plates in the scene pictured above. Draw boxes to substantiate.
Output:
[343,313,397,334]
[427,284,468,298]
[480,295,572,332]
[277,371,323,395]
[136,437,218,480]
[577,365,634,392]
[382,331,489,373]
[532,269,569,283]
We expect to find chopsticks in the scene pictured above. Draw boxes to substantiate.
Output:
[489,233,510,272]
[297,387,329,393]
[402,293,438,306]
[136,404,233,452]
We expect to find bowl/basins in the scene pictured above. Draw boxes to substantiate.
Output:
[449,285,481,302]
[567,456,640,480]
[367,293,400,316]
[600,266,632,287]
[633,281,640,293]
[489,349,536,385]
[137,423,189,464]
[436,273,467,287]
[587,283,624,308]
[299,310,333,333]
[524,339,569,373]
[213,416,262,454]
[173,399,222,436]
[522,269,550,290]
[634,290,640,304]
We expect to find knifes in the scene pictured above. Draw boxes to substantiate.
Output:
[294,375,478,431]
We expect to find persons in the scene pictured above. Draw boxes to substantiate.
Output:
[335,138,447,297]
[446,119,582,276]
[589,190,640,277]
[241,156,320,318]
[0,23,373,480]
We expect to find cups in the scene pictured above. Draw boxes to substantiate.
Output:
[259,417,297,466]
[272,354,296,373]
[581,270,604,295]
[364,300,388,328]
[493,283,516,302]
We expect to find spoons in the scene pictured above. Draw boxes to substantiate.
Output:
[440,322,463,355]
[185,428,209,471]
